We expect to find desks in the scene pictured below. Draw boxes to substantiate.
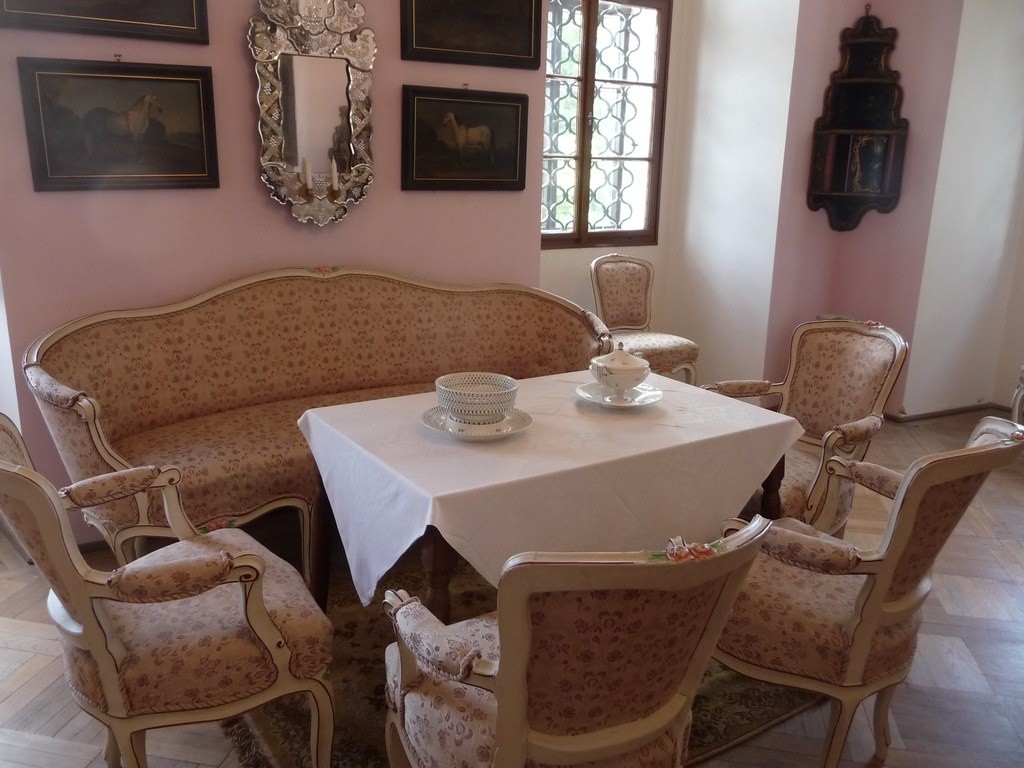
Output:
[295,369,806,704]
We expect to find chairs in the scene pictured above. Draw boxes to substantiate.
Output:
[589,253,700,388]
[707,415,1024,768]
[696,317,910,542]
[383,513,774,768]
[0,413,339,768]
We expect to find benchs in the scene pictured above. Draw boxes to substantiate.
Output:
[22,265,616,592]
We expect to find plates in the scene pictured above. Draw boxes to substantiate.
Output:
[576,380,664,409]
[420,405,534,442]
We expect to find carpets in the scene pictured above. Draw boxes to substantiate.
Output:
[219,511,832,768]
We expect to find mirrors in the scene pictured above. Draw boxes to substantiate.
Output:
[246,0,376,229]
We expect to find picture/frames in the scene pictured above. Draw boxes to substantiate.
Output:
[399,0,543,70]
[0,0,210,45]
[402,84,528,191]
[15,57,218,191]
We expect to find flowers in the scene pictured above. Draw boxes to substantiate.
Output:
[305,266,341,276]
